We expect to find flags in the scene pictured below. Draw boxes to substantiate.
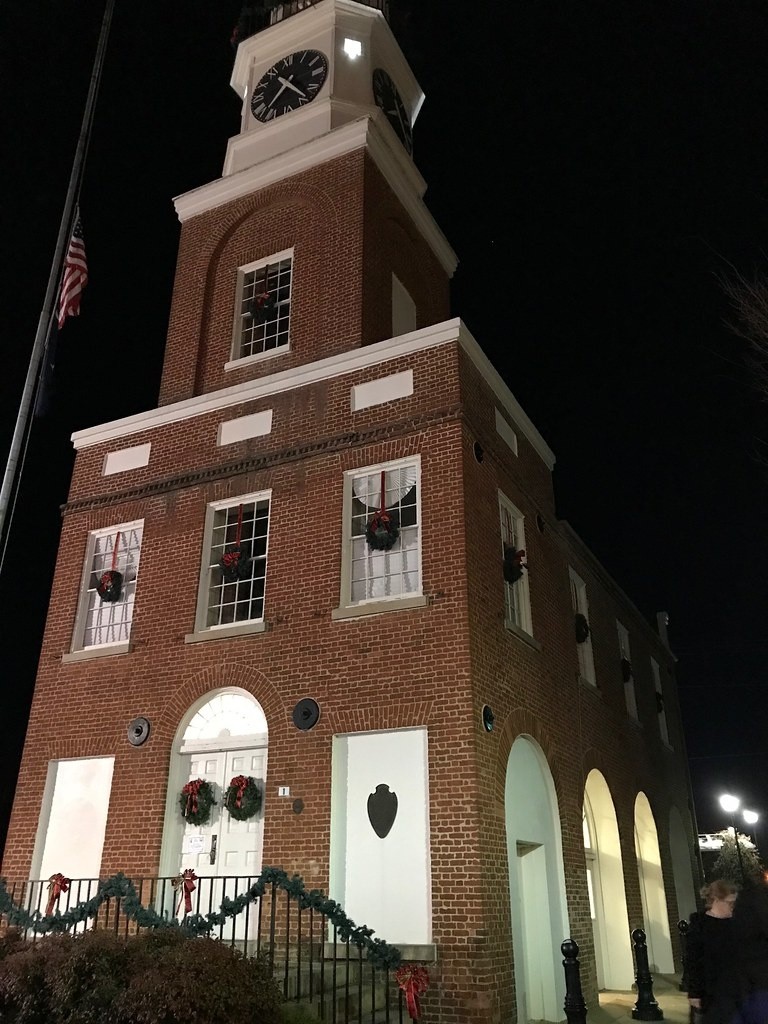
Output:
[55,205,88,330]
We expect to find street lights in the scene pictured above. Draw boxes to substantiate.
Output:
[717,788,761,884]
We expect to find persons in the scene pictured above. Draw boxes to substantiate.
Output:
[683,814,768,1024]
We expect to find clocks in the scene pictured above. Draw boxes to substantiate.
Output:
[372,68,413,156]
[250,49,328,123]
